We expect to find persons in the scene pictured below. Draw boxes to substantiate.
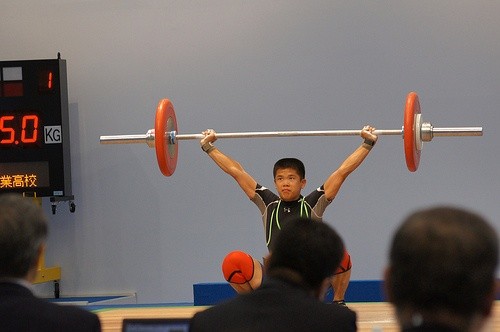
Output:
[189,217,358,332]
[382,206,500,332]
[199,125,379,306]
[0,195,101,332]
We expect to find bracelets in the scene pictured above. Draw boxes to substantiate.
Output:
[201,142,216,154]
[361,138,376,150]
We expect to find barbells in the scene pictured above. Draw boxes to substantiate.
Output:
[97,92,483,177]
